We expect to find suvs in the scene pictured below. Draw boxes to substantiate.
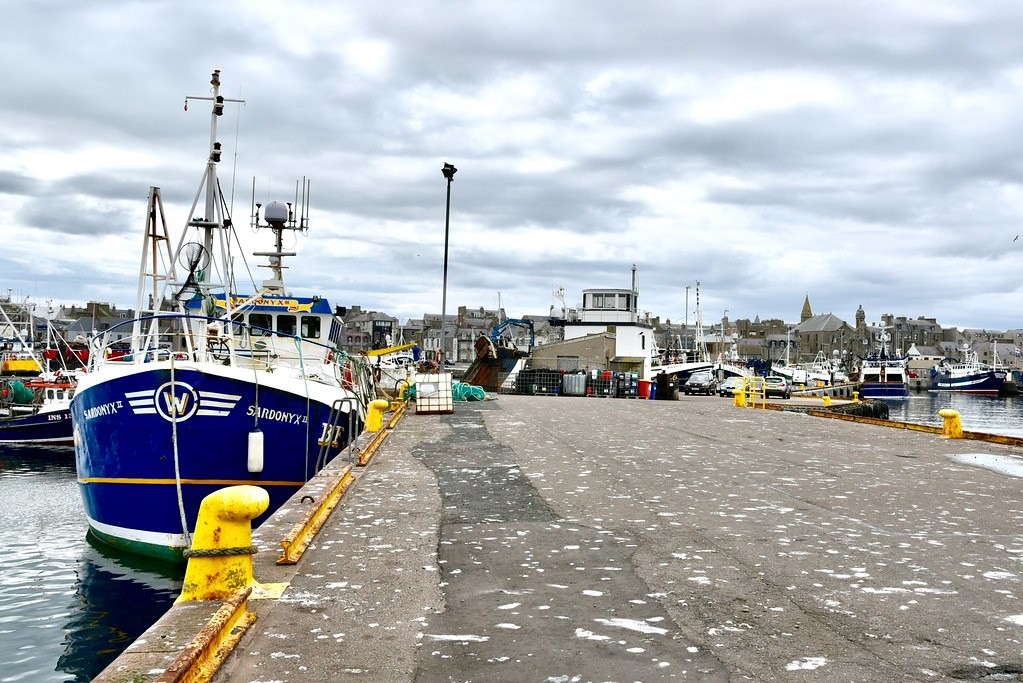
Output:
[761,376,792,399]
[684,372,718,396]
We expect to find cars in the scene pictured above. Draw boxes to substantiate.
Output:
[720,377,750,397]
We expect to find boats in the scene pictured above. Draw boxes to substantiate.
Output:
[714,331,1023,401]
[0,70,420,585]
[53,522,187,683]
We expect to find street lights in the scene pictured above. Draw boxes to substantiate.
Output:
[839,328,844,360]
[685,284,692,349]
[438,160,459,376]
[772,324,777,341]
[724,309,730,339]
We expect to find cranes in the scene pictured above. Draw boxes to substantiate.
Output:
[491,317,534,354]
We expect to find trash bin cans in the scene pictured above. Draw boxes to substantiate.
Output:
[638,379,658,400]
